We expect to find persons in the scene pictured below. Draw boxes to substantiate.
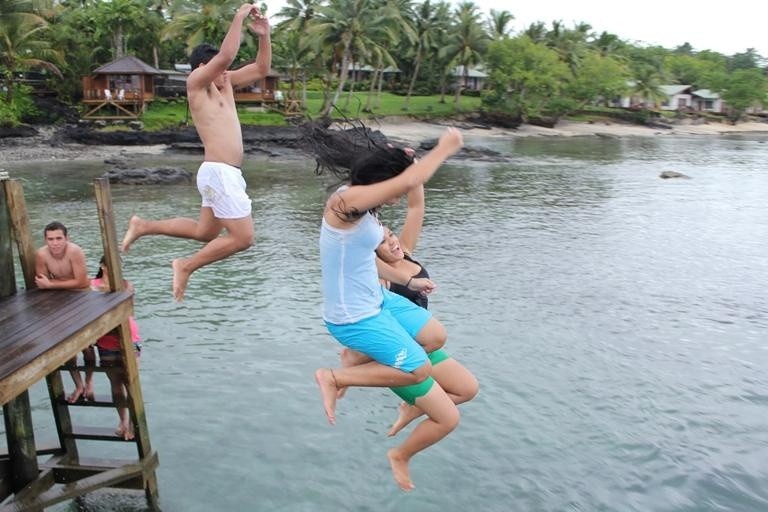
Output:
[117,1,273,303]
[374,148,480,492]
[88,253,142,441]
[34,220,97,404]
[281,95,469,427]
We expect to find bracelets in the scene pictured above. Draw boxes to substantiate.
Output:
[404,275,414,289]
